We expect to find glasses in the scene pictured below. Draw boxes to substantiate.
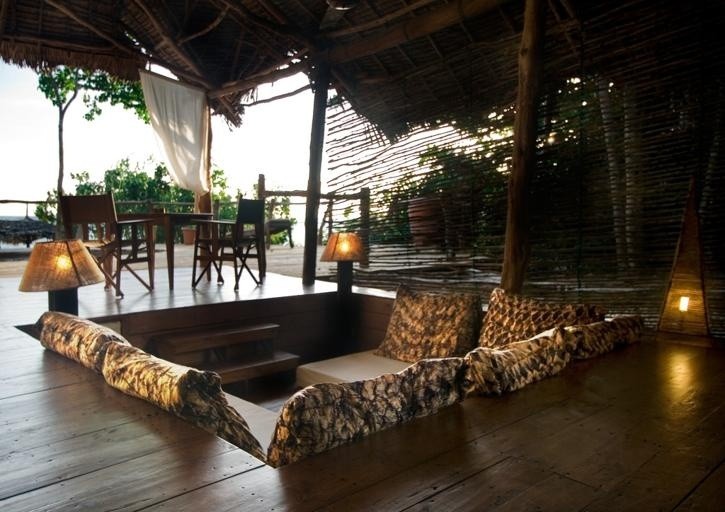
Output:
[320,232,367,321]
[18,237,106,317]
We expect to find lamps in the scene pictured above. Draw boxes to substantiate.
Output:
[18,237,106,317]
[58,189,267,300]
[320,232,367,321]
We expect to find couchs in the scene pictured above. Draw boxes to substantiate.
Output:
[41,285,644,469]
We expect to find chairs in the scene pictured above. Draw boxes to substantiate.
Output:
[58,189,267,300]
